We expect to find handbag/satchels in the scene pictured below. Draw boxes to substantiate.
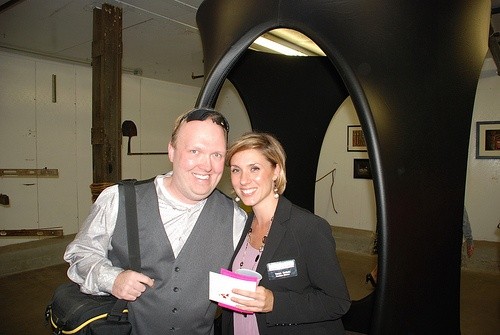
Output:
[45,284,132,335]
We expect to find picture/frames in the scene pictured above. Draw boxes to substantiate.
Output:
[476,120,500,160]
[353,159,373,179]
[347,125,368,152]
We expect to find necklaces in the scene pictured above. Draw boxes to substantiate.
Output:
[239,216,274,270]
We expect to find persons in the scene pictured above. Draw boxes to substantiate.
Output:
[63,108,248,335]
[222,133,352,335]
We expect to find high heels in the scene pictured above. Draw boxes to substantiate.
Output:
[365,273,376,288]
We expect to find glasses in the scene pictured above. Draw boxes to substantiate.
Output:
[171,108,230,136]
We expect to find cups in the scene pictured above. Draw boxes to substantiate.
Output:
[235,269,263,286]
